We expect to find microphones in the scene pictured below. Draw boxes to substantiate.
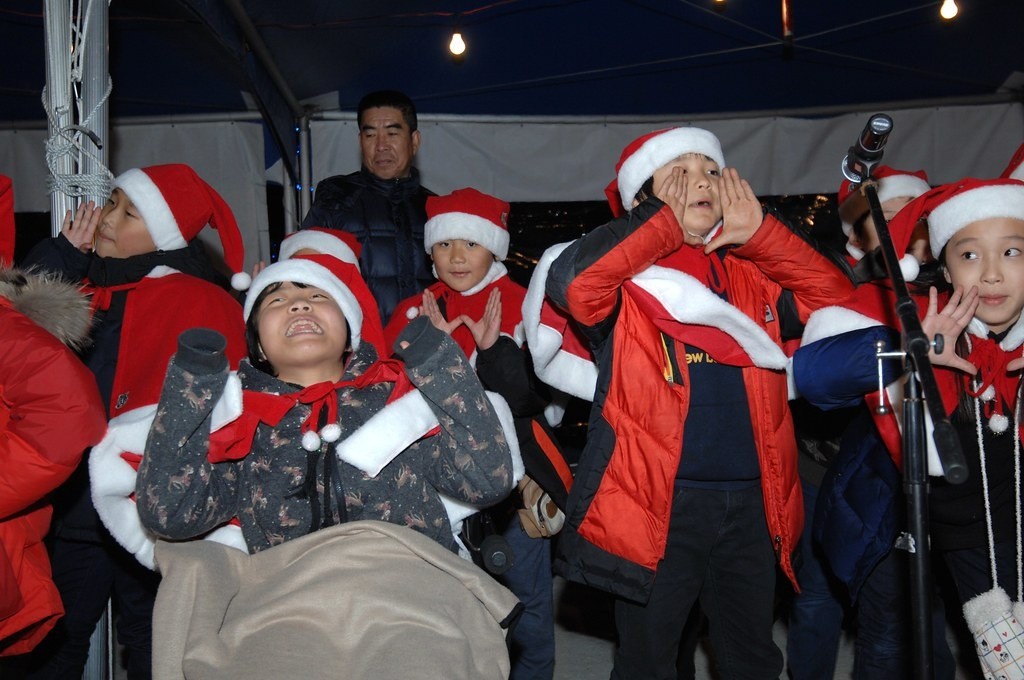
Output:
[842,113,893,183]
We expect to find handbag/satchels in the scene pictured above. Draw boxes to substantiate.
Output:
[517,474,566,539]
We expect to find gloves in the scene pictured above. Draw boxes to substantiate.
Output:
[963,586,1024,680]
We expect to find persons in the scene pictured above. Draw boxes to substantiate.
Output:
[382,188,572,680]
[788,147,1024,680]
[522,126,802,680]
[89,226,524,680]
[0,175,107,680]
[302,89,437,326]
[23,162,248,680]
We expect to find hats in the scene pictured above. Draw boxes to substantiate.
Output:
[999,143,1024,181]
[424,187,511,261]
[886,177,1024,282]
[278,227,362,276]
[604,126,726,219]
[244,253,387,362]
[108,163,252,291]
[837,164,932,237]
